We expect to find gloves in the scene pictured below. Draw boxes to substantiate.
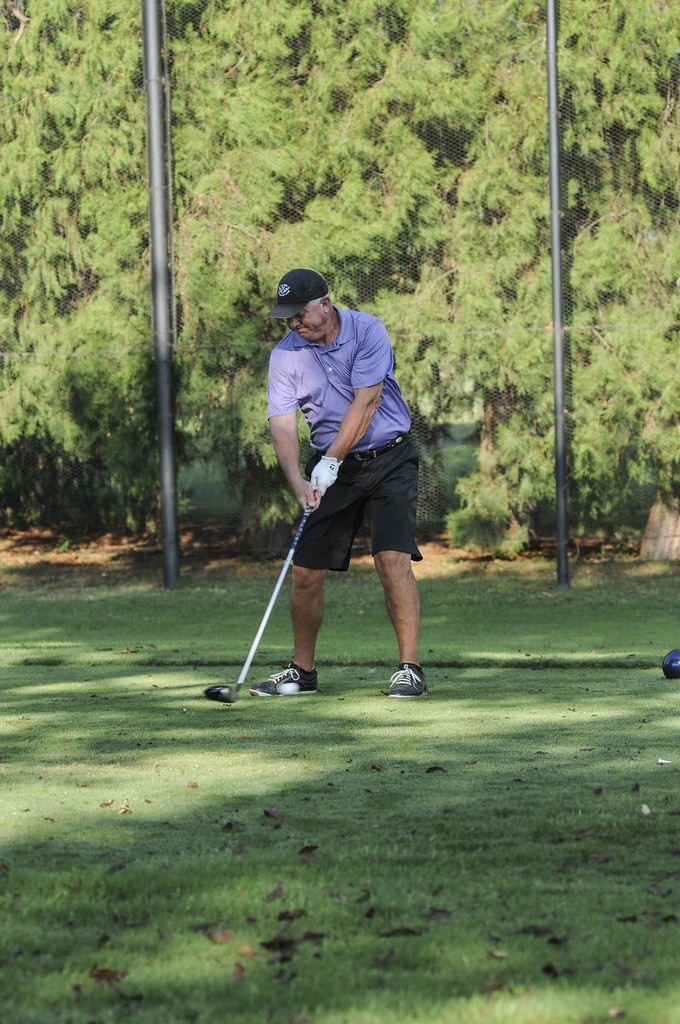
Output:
[310,454,342,496]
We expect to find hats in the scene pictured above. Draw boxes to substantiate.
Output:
[266,267,330,318]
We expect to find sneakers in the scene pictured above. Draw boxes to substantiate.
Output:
[249,660,317,697]
[388,664,427,698]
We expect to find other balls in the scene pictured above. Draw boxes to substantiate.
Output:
[662,649,680,679]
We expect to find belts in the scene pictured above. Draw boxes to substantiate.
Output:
[343,432,411,461]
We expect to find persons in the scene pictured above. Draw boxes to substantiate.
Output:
[248,268,427,698]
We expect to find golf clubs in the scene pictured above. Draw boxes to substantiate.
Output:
[203,489,319,703]
